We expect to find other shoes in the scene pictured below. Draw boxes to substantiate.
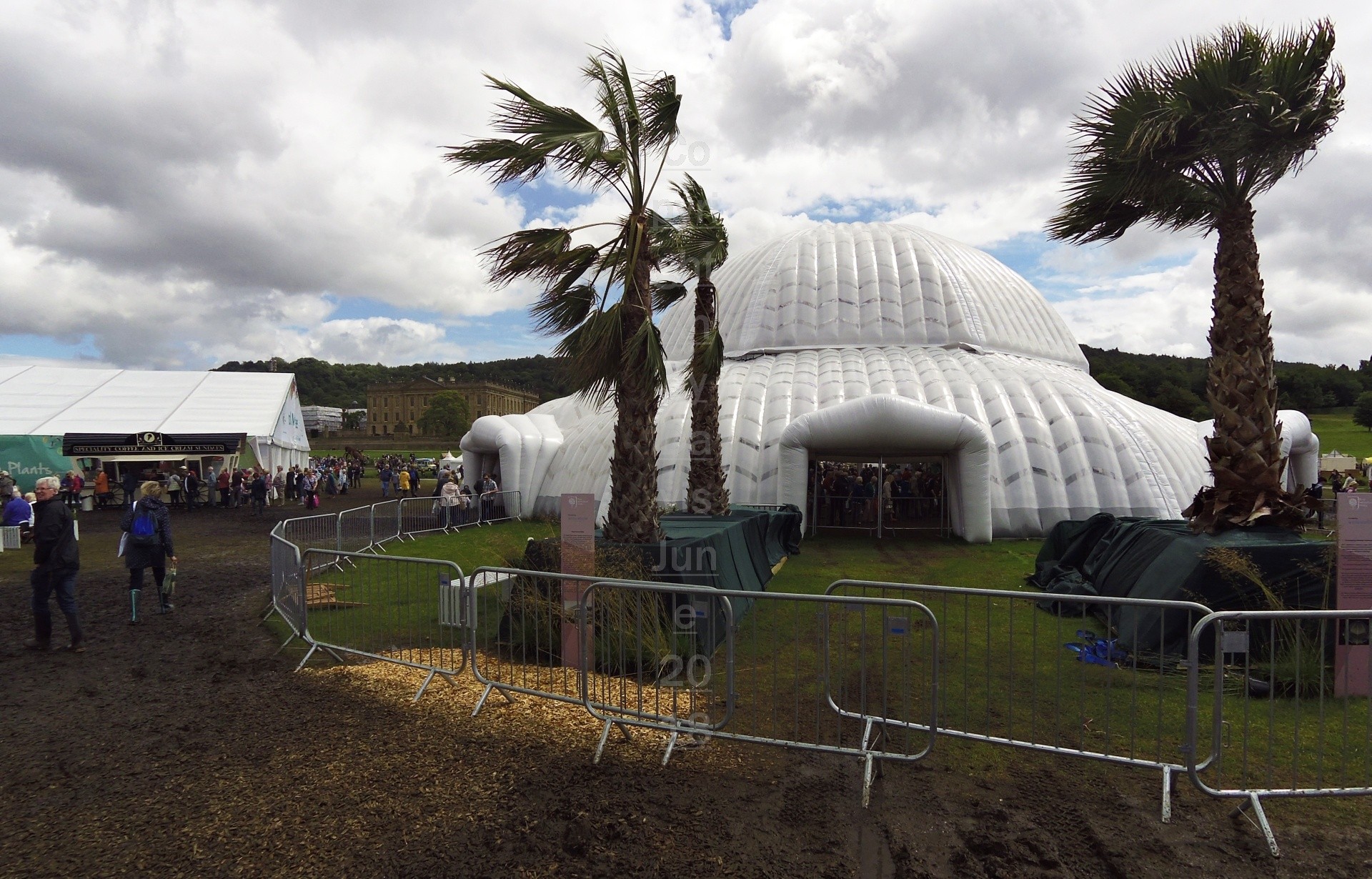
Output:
[264,505,269,508]
[251,513,256,515]
[276,498,293,506]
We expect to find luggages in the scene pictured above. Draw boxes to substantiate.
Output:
[305,494,319,508]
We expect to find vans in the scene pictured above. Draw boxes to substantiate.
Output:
[411,458,437,471]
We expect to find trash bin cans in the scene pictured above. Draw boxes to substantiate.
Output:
[81,495,93,510]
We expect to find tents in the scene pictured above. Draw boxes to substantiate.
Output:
[0,366,311,507]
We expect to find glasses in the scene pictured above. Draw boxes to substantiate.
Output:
[35,487,56,490]
[484,478,488,480]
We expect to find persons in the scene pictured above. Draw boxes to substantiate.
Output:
[55,470,85,511]
[1329,469,1358,507]
[207,466,217,507]
[122,467,138,510]
[119,481,178,623]
[807,460,946,529]
[1305,476,1329,530]
[0,471,38,543]
[22,476,87,652]
[376,452,420,499]
[217,454,366,516]
[1356,463,1368,477]
[149,469,180,510]
[179,471,204,511]
[432,461,498,533]
[93,468,109,510]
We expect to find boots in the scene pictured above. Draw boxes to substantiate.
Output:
[157,586,175,614]
[128,589,144,625]
[298,496,304,505]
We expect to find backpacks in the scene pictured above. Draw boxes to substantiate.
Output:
[130,501,161,547]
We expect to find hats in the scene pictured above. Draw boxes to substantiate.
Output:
[179,466,187,469]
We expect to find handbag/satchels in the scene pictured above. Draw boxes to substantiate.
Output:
[190,490,199,498]
[117,531,130,559]
[162,563,176,595]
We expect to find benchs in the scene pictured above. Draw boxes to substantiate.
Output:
[438,570,518,630]
[0,524,35,549]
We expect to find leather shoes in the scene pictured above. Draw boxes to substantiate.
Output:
[23,638,49,649]
[62,643,86,653]
